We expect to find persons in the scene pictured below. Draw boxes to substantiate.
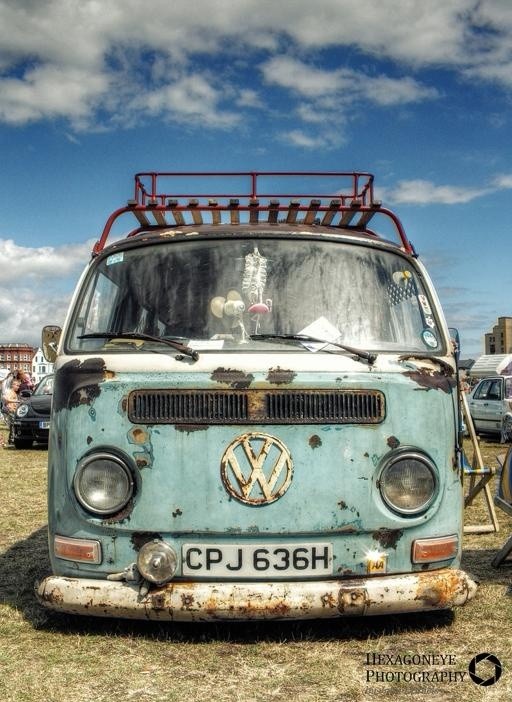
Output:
[1,380,23,446]
[15,371,38,394]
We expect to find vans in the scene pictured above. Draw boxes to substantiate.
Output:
[35,170,478,624]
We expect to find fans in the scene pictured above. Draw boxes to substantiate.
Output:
[210,290,251,345]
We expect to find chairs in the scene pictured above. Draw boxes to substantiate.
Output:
[458,381,500,535]
[488,445,512,570]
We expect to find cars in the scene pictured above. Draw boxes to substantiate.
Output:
[465,374,511,445]
[9,370,53,449]
